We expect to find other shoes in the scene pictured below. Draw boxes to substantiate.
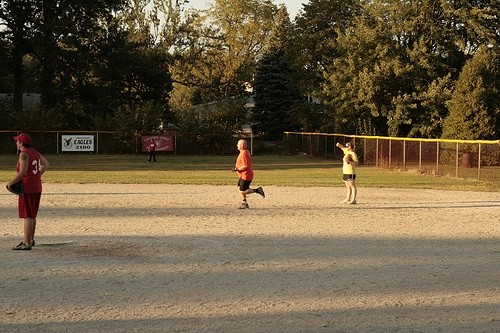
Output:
[238,203,249,209]
[341,200,351,204]
[11,242,32,250]
[256,187,265,198]
[347,200,356,205]
[32,240,35,246]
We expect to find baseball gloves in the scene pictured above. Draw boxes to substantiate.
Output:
[6,179,25,197]
[344,153,354,165]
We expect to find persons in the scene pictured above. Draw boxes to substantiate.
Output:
[6,133,49,249]
[336,141,359,205]
[233,139,266,208]
[148,138,157,162]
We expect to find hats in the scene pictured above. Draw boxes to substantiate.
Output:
[13,133,33,145]
